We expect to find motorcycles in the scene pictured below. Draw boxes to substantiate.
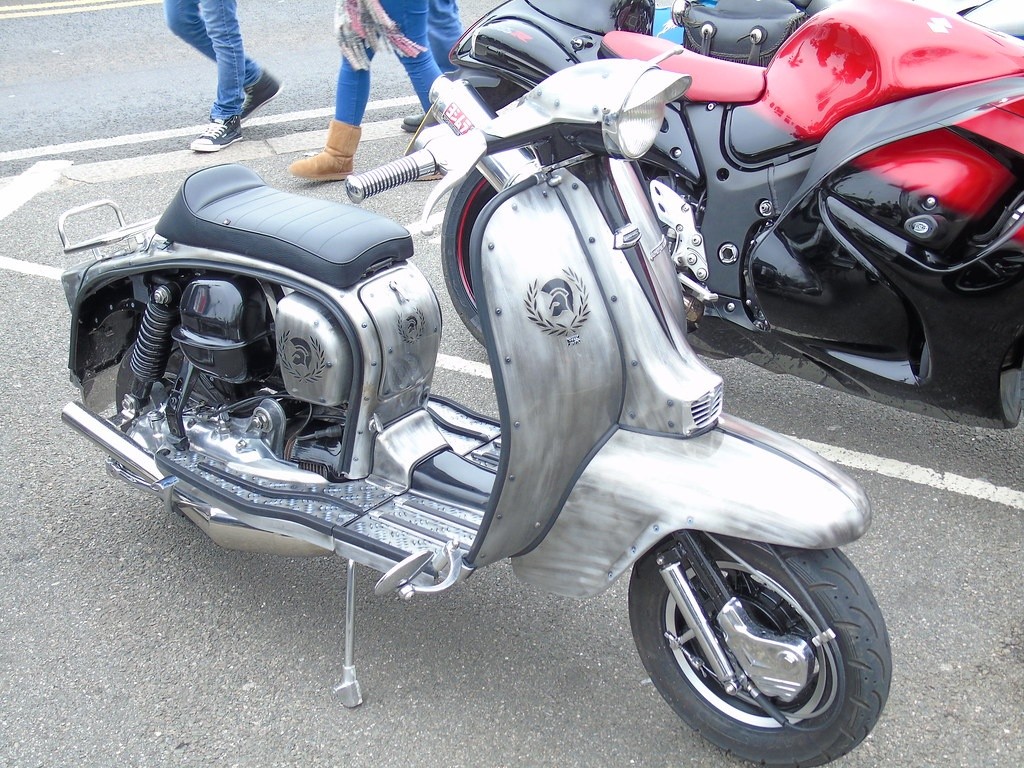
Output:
[663,1,1024,68]
[403,1,1024,431]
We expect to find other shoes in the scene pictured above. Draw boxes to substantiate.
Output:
[401,113,426,133]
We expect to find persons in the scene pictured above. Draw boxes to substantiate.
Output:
[164,0,284,150]
[287,0,445,182]
[401,0,464,132]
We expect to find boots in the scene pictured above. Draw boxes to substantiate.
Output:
[287,118,361,182]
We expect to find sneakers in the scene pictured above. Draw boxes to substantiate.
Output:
[239,69,282,124]
[191,115,244,153]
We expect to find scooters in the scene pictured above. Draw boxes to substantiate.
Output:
[54,49,895,768]
